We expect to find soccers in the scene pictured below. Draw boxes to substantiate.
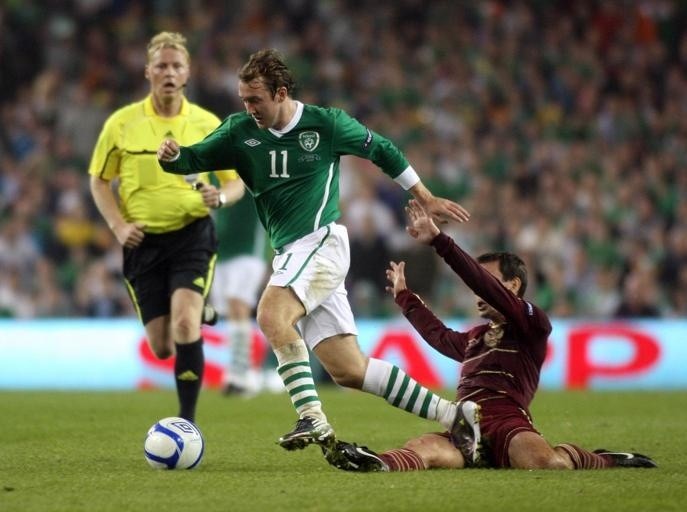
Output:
[144,417,204,470]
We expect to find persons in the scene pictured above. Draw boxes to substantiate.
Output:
[87,31,246,427]
[209,191,266,396]
[0,1,686,320]
[157,48,484,470]
[323,199,661,474]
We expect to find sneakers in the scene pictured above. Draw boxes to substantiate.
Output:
[592,449,631,468]
[449,400,483,468]
[202,303,219,326]
[320,438,389,473]
[273,415,336,452]
